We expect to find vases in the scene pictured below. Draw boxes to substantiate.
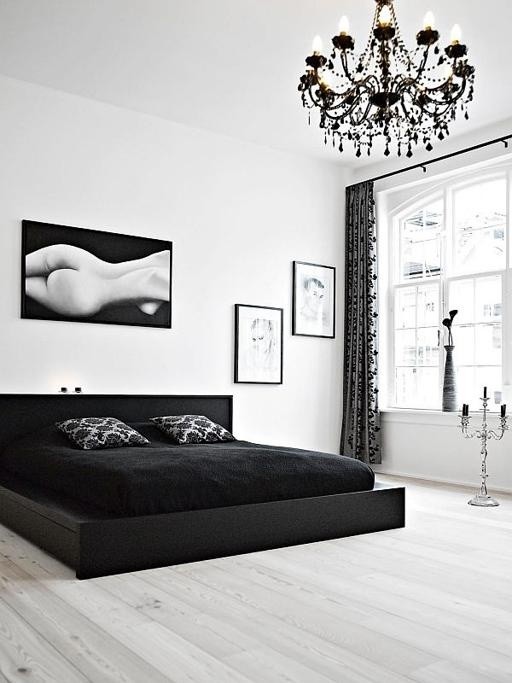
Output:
[442,344,459,412]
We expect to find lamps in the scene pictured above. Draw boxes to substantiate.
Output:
[293,1,478,161]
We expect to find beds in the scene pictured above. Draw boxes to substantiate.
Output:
[1,392,409,581]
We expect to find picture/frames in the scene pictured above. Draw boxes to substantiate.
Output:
[20,216,175,330]
[290,261,338,340]
[233,302,285,385]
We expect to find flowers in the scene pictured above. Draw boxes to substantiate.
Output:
[442,310,460,345]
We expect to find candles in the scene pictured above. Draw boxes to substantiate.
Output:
[482,386,487,398]
[500,403,507,417]
[461,404,469,416]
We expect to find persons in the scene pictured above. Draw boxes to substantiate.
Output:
[297,277,329,323]
[248,315,277,357]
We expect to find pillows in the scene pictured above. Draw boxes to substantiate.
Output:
[52,410,236,450]
